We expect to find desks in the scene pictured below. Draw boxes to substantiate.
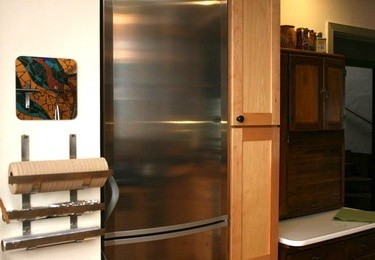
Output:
[278,207,375,259]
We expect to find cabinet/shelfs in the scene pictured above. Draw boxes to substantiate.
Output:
[279,48,346,221]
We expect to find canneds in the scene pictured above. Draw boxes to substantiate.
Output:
[297,28,308,50]
[315,37,326,53]
[280,25,296,48]
[316,32,323,40]
[308,30,316,51]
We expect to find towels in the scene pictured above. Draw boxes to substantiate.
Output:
[333,209,375,223]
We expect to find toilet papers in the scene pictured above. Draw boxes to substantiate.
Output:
[7,157,109,194]
